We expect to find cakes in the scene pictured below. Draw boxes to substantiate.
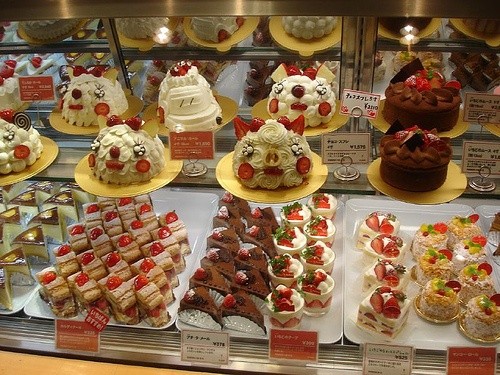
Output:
[14,15,500,43]
[0,59,462,192]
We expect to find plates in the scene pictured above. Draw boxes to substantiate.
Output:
[410,238,500,344]
[0,87,470,206]
[15,18,500,59]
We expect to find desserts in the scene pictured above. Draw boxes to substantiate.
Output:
[0,179,500,344]
[0,28,500,107]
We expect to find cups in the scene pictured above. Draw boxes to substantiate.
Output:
[264,193,338,329]
